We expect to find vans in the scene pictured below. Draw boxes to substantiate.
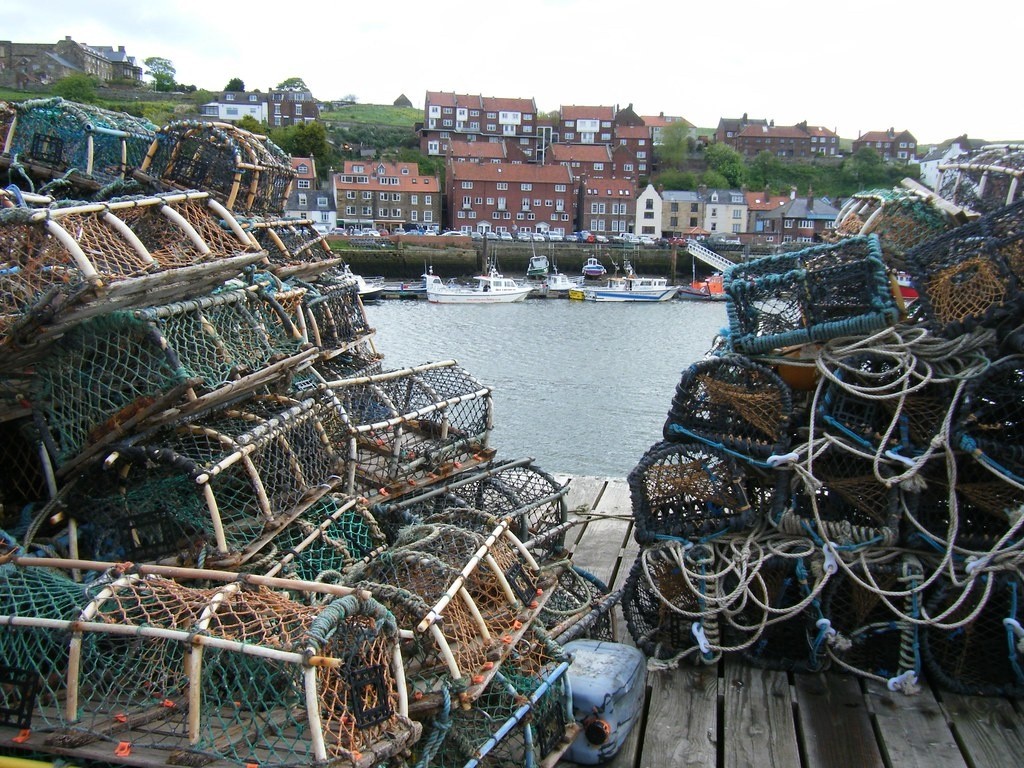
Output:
[577,231,593,243]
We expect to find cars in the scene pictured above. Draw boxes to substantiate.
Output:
[596,236,609,243]
[612,233,667,247]
[379,229,389,236]
[332,228,347,236]
[407,230,422,235]
[442,232,462,236]
[499,232,513,242]
[487,233,498,241]
[318,230,327,236]
[513,233,532,242]
[531,233,545,241]
[668,237,686,247]
[472,232,483,241]
[566,236,577,242]
[346,229,363,236]
[424,230,436,236]
[544,231,563,241]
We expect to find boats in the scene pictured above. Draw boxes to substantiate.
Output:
[678,273,733,300]
[426,266,534,304]
[518,276,549,296]
[582,258,607,278]
[549,266,576,294]
[889,271,918,298]
[526,256,550,282]
[569,259,681,301]
[343,264,384,303]
